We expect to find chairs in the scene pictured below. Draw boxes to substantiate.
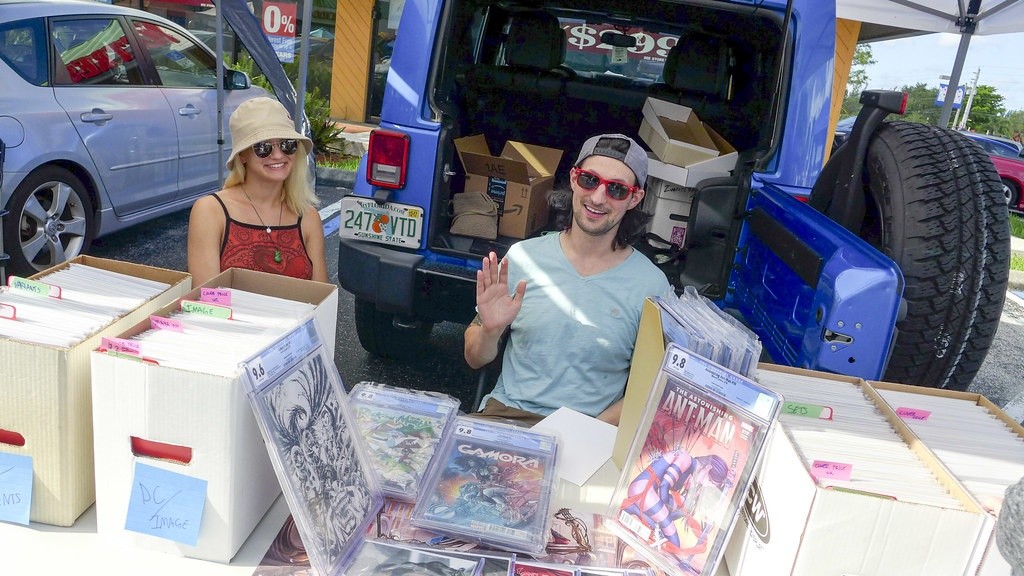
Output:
[473,10,737,107]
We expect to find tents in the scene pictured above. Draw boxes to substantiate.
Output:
[836,0,1024,37]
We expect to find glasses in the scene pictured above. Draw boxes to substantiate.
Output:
[252,139,300,159]
[575,166,639,200]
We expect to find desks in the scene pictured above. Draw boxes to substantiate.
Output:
[0,384,1024,576]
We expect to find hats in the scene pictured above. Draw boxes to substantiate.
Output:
[226,97,313,170]
[575,134,648,190]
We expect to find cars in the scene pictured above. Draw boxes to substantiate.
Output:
[0,1,314,281]
[828,110,859,156]
[334,1,1011,398]
[956,131,1024,216]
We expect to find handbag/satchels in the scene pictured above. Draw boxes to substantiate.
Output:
[632,232,689,296]
[447,192,497,240]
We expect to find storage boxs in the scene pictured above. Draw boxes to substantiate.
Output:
[0,250,193,530]
[449,126,559,242]
[85,260,341,566]
[723,358,1024,576]
[639,92,741,267]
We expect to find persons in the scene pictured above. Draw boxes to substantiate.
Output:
[995,475,1024,576]
[464,133,671,426]
[969,126,978,134]
[186,97,328,292]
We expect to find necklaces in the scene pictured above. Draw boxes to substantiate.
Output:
[241,182,284,262]
